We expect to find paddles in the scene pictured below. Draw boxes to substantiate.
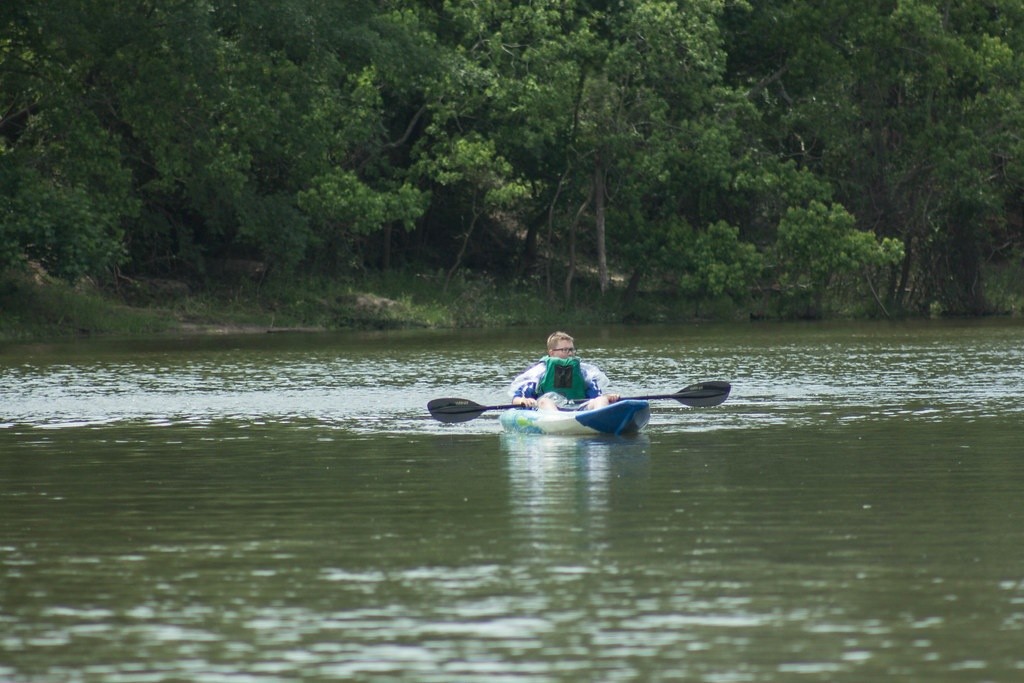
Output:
[427,379,731,424]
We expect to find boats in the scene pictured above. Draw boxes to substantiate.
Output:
[498,397,652,435]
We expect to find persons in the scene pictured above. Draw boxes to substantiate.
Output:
[507,330,620,410]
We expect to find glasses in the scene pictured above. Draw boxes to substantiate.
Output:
[552,347,577,354]
